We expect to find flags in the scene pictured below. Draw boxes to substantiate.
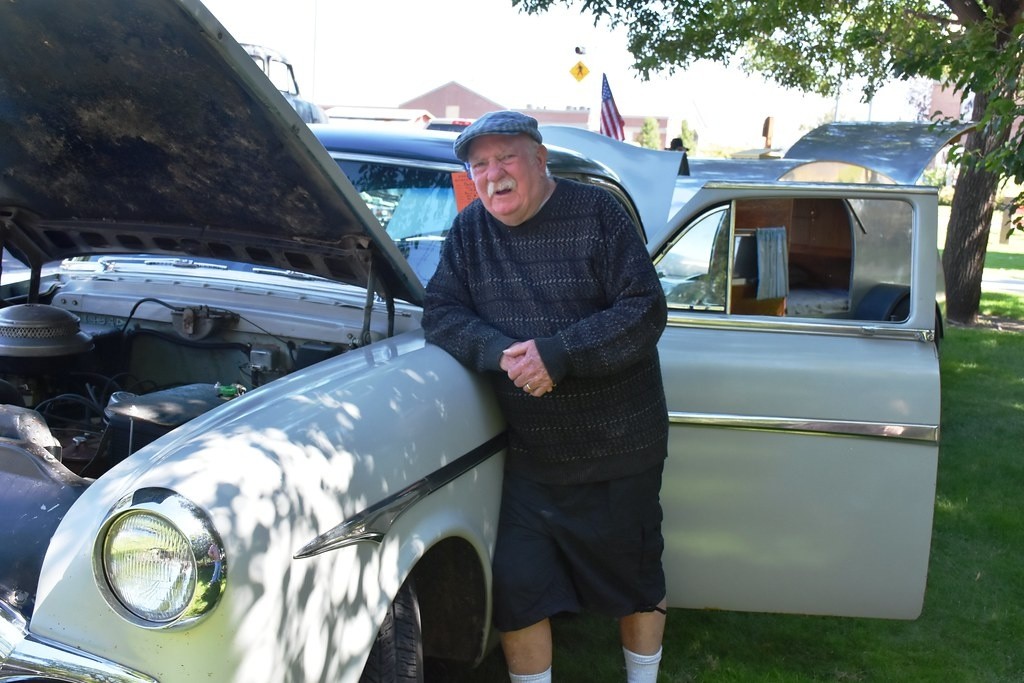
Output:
[600,73,625,141]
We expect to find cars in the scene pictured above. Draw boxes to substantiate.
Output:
[325,107,473,140]
[0,0,984,683]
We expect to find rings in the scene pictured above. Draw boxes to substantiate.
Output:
[526,384,534,392]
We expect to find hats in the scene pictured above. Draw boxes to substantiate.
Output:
[453,111,543,161]
[664,138,689,152]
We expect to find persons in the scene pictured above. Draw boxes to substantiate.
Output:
[420,110,670,683]
[664,137,690,176]
